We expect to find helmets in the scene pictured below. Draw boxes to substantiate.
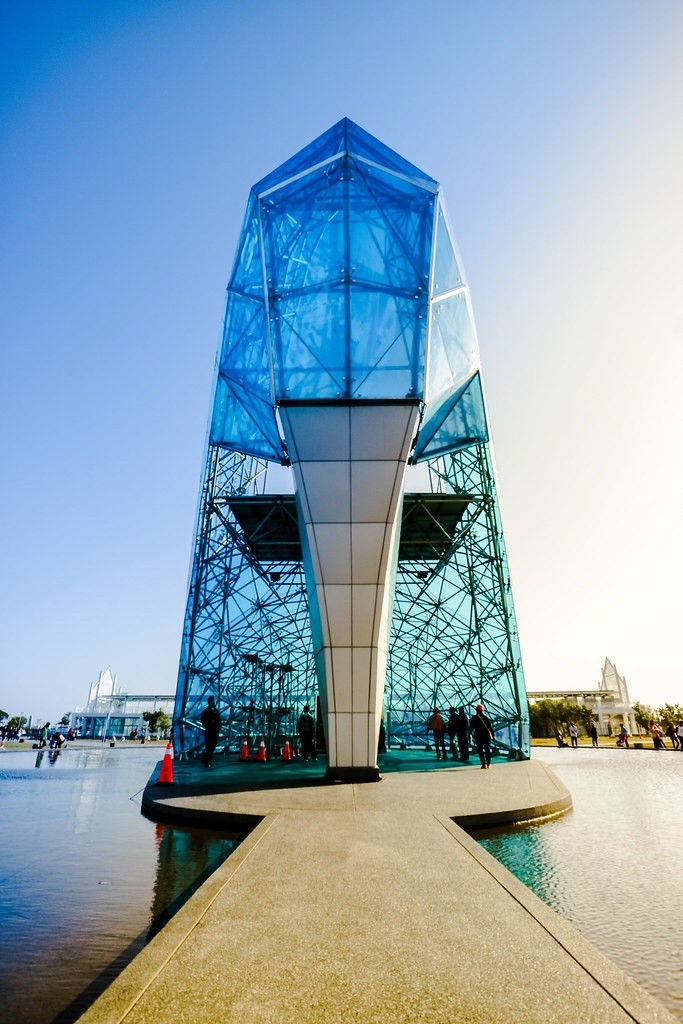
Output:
[570,721,574,725]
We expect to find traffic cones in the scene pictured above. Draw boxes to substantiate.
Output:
[240,737,249,760]
[158,743,174,783]
[283,738,291,760]
[255,738,266,761]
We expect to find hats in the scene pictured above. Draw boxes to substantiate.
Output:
[433,707,441,714]
[474,704,484,711]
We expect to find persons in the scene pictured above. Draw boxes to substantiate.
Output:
[469,704,495,769]
[201,696,221,768]
[130,729,137,741]
[141,727,145,743]
[649,720,683,749]
[162,728,166,739]
[447,706,458,760]
[569,721,578,746]
[296,706,318,762]
[2,726,23,741]
[39,722,76,749]
[619,722,629,747]
[428,707,448,759]
[556,730,569,746]
[590,724,598,746]
[456,706,469,761]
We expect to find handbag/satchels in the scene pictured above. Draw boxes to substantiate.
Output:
[675,725,679,733]
[489,730,493,740]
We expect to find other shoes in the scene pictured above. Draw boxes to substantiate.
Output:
[571,745,578,747]
[451,756,469,761]
[302,756,320,762]
[436,754,448,760]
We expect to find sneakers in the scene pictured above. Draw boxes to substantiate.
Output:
[481,763,491,769]
